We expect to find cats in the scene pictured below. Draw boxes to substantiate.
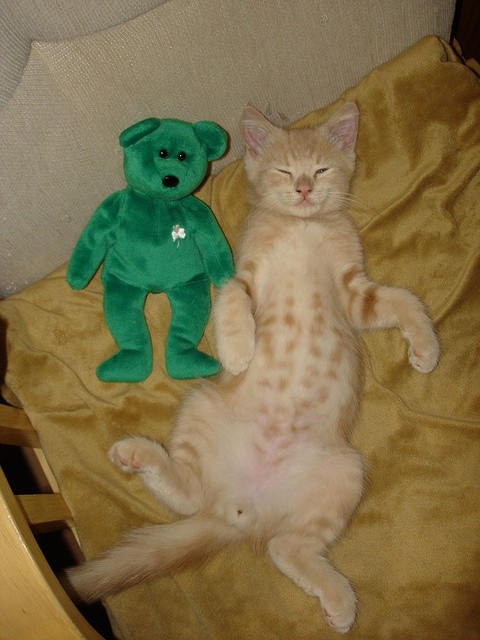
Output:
[53,100,441,634]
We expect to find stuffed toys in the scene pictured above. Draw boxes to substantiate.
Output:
[65,117,237,384]
